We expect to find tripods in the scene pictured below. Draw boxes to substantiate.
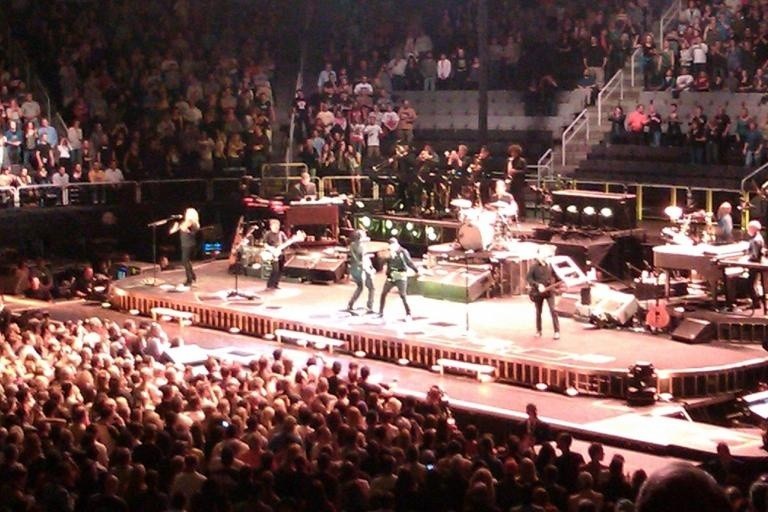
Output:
[145,224,170,288]
[226,254,253,300]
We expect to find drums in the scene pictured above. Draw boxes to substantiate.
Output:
[480,212,497,225]
[459,220,493,251]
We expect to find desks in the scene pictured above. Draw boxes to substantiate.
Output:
[548,186,639,229]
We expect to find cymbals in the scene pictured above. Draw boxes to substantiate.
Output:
[450,198,472,207]
[490,201,508,207]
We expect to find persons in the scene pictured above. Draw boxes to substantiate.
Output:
[0,1,766,241]
[166,206,201,288]
[1,310,768,512]
[343,213,379,314]
[375,234,422,319]
[521,248,566,340]
[741,221,766,313]
[257,218,294,290]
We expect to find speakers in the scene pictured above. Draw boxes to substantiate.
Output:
[283,254,318,281]
[552,296,578,318]
[672,317,713,344]
[310,257,346,283]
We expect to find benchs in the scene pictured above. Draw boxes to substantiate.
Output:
[386,46,768,213]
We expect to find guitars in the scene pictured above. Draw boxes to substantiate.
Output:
[258,230,306,263]
[529,278,565,302]
[645,269,671,333]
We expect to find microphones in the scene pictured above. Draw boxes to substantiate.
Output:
[170,214,182,219]
[249,225,259,230]
[464,249,473,254]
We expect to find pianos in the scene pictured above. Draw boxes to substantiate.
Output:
[651,242,752,309]
